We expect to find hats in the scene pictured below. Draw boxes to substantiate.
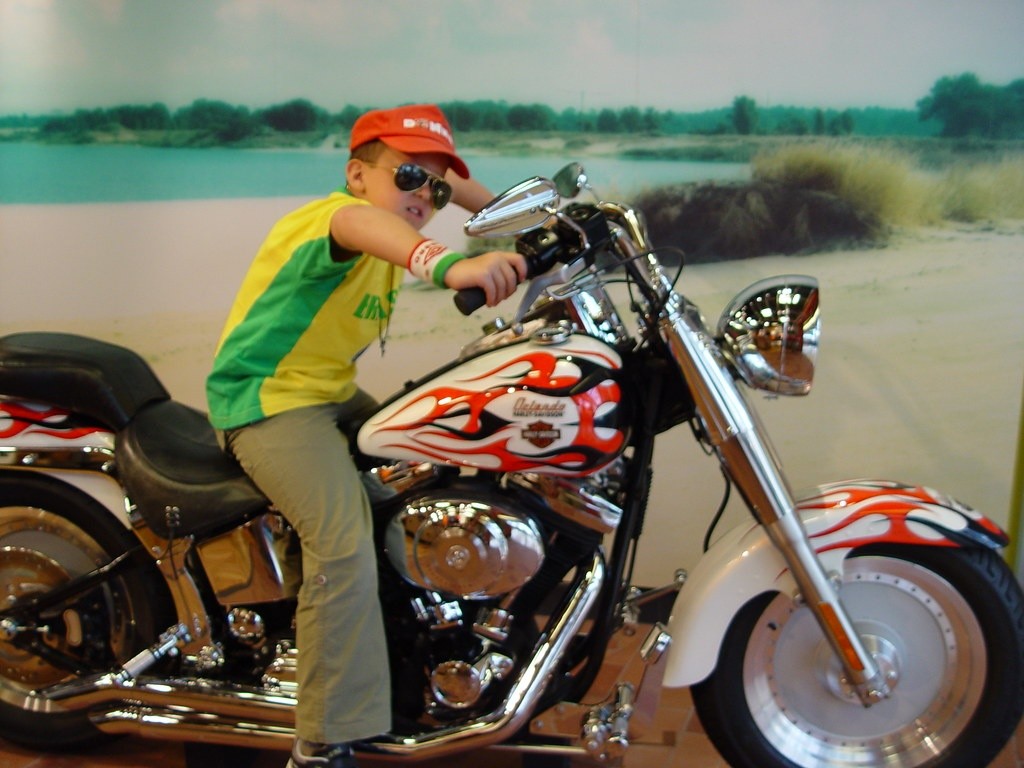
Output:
[350,104,470,179]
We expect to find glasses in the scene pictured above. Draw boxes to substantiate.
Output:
[364,160,452,210]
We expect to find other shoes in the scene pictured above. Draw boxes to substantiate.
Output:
[286,735,360,768]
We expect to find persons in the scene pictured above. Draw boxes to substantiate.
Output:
[201,105,529,768]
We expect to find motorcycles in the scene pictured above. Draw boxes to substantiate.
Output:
[1,163,1024,768]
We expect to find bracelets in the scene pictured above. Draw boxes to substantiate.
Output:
[405,241,467,289]
